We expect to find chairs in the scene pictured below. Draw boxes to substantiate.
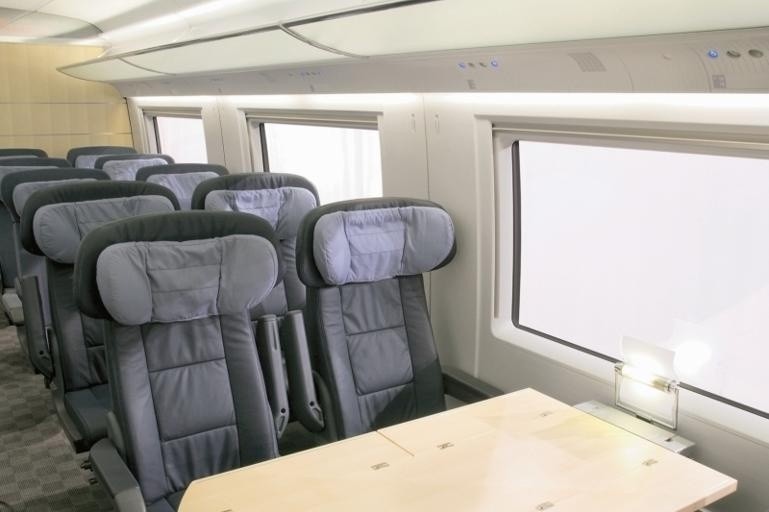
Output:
[0,145,233,391]
[70,208,291,512]
[190,172,322,394]
[283,196,460,443]
[18,179,185,455]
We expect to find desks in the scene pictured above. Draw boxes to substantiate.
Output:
[175,387,741,512]
